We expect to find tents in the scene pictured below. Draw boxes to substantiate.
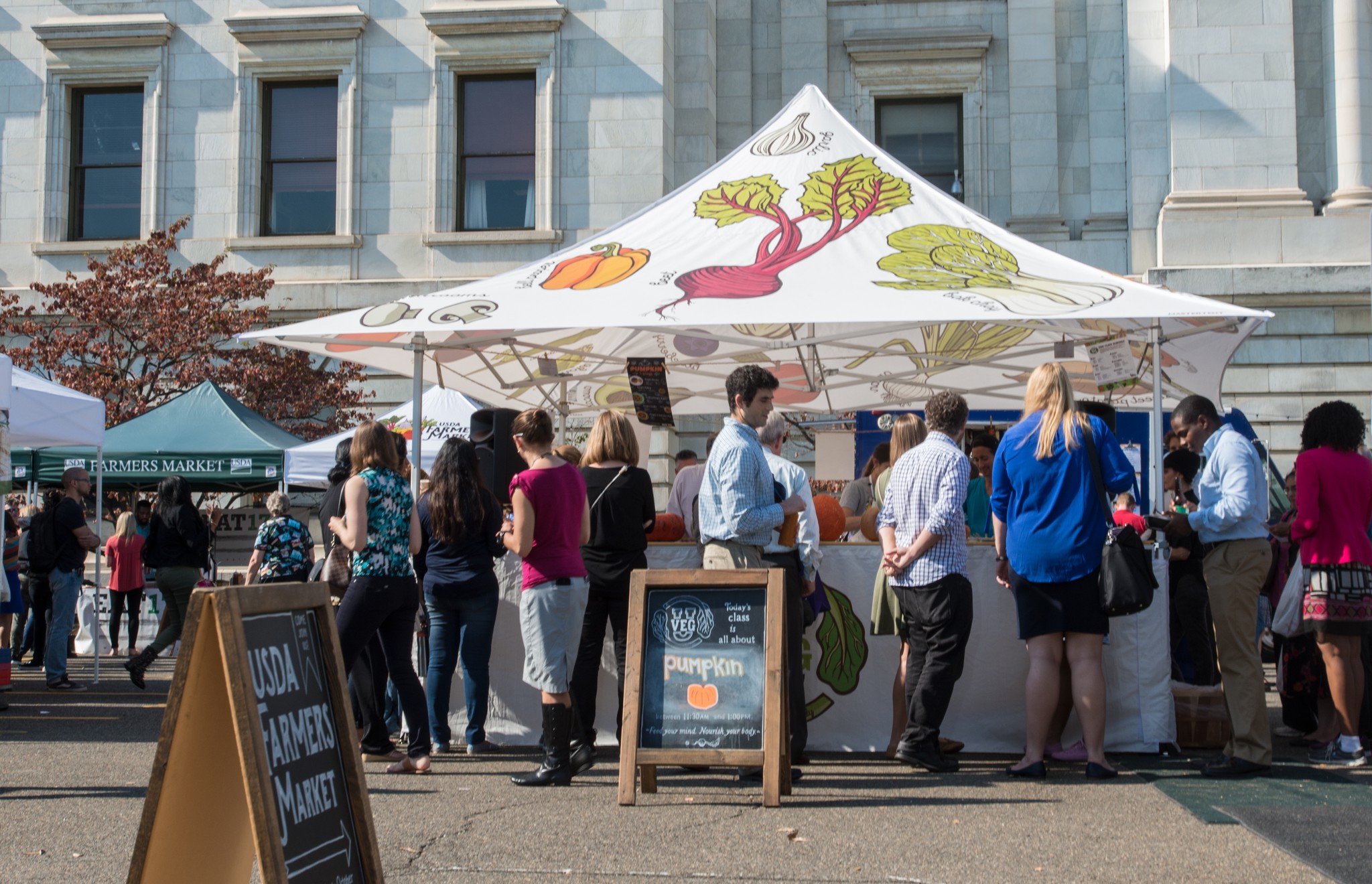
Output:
[1,80,1273,683]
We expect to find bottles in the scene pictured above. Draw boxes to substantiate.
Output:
[778,512,798,548]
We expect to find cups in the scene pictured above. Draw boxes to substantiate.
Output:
[1174,495,1189,514]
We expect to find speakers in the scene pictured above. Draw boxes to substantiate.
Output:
[469,407,530,504]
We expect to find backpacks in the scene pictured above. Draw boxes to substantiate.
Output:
[27,501,83,573]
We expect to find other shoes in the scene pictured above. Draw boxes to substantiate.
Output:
[886,745,899,759]
[936,737,964,751]
[793,755,810,765]
[1199,758,1273,778]
[740,767,804,781]
[1085,761,1119,781]
[466,741,500,753]
[432,741,449,754]
[1005,761,1046,780]
[362,749,406,763]
[1274,725,1307,738]
[894,749,960,771]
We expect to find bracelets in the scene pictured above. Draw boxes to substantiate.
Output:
[996,556,1007,561]
[211,521,218,527]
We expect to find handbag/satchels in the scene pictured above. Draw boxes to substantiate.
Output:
[1272,553,1312,638]
[1099,527,1157,617]
[319,550,353,589]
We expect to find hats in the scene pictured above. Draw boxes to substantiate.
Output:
[1163,449,1201,483]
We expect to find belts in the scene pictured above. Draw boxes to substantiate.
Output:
[1201,540,1219,556]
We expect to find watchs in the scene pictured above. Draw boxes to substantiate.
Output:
[496,531,510,546]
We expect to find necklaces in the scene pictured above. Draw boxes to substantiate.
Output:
[532,453,552,466]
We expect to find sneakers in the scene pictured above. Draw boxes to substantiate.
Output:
[17,660,45,669]
[1309,738,1368,767]
[48,672,87,692]
[1054,739,1086,762]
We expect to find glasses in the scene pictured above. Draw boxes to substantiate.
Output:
[71,478,91,483]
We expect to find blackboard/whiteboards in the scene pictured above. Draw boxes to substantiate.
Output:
[122,578,383,883]
[615,562,794,764]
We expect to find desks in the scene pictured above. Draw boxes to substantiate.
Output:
[74,587,180,656]
[450,541,1175,760]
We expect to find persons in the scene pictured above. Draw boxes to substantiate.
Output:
[1,356,1371,787]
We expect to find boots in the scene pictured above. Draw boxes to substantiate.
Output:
[124,644,159,689]
[569,708,594,774]
[510,702,576,786]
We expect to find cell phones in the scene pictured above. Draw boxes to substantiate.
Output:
[1147,516,1170,531]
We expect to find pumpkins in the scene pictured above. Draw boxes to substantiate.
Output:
[811,493,846,541]
[645,513,685,542]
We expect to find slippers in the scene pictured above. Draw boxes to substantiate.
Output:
[386,758,434,775]
